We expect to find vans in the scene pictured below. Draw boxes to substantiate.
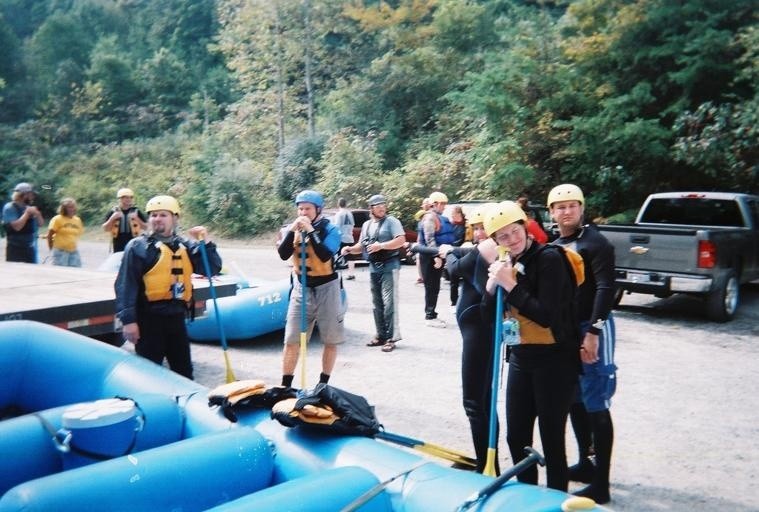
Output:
[524,202,598,239]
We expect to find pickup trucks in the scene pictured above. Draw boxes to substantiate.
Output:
[589,189,759,323]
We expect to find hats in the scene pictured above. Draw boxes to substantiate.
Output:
[13,182,40,196]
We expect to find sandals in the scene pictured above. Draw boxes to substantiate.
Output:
[367,337,396,352]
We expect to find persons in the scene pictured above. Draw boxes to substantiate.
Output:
[332,196,356,280]
[114,196,222,381]
[276,189,347,387]
[415,191,548,330]
[542,183,615,503]
[408,204,502,477]
[477,199,579,493]
[340,196,406,352]
[1,183,45,264]
[46,198,86,265]
[101,188,147,251]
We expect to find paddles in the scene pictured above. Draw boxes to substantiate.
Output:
[375,431,477,467]
[300,225,305,389]
[482,246,511,478]
[198,234,237,383]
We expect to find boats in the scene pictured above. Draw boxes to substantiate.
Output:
[1,317,613,511]
[98,245,350,347]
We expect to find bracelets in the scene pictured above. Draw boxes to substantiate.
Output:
[379,242,385,250]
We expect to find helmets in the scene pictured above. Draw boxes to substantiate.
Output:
[547,184,584,206]
[470,201,527,236]
[369,195,387,206]
[144,195,180,214]
[295,190,323,207]
[117,189,134,198]
[430,192,448,202]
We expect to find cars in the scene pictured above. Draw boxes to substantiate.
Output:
[276,207,420,267]
